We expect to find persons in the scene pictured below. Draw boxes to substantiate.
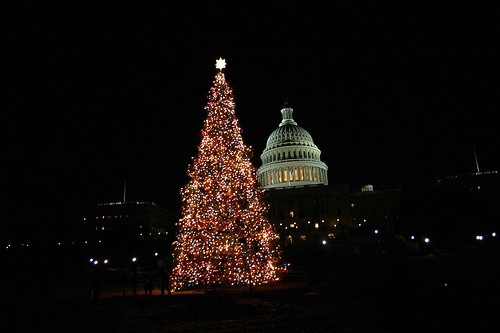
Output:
[131,263,141,296]
[143,271,154,296]
[159,267,172,298]
